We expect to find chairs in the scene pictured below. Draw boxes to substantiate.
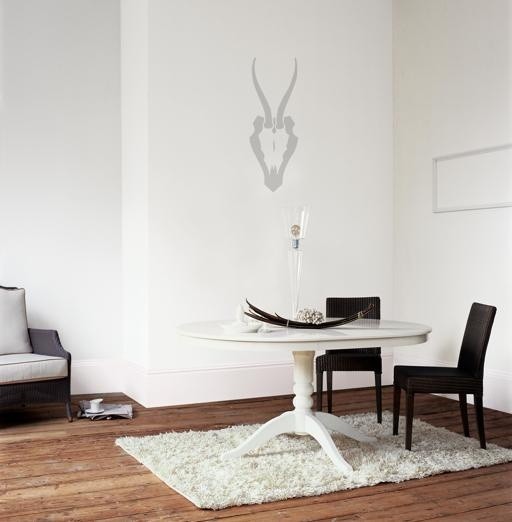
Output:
[0,281,73,429]
[394,298,499,452]
[313,296,389,426]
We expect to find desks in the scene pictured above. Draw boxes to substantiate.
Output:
[178,316,431,479]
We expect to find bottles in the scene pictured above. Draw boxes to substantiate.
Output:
[236,302,245,324]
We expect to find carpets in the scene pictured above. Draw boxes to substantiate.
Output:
[114,405,511,511]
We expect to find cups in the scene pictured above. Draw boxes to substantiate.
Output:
[90,399,104,412]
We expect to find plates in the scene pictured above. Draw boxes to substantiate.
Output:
[86,408,104,413]
[218,321,261,333]
